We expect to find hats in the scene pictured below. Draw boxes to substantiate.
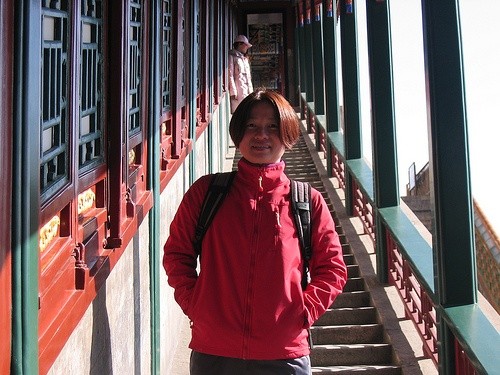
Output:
[234,34,253,48]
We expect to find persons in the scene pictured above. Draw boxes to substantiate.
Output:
[160,86,348,375]
[228,33,256,115]
